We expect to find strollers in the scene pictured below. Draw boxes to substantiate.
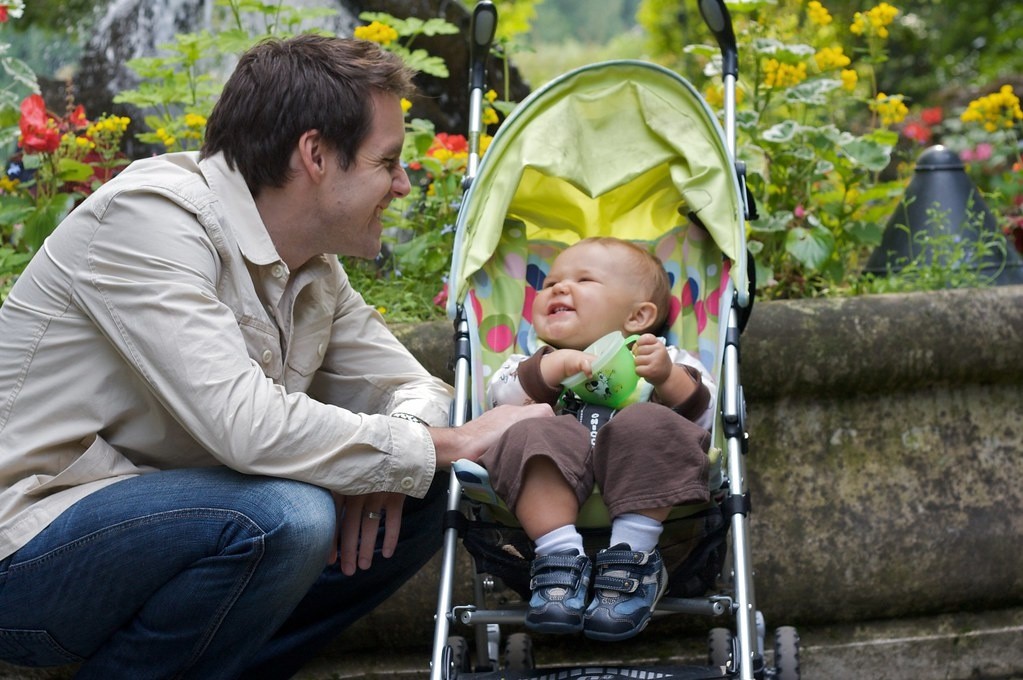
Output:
[427,1,800,680]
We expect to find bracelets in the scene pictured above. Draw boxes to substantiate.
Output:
[392,413,421,423]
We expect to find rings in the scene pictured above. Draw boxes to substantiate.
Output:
[365,512,381,519]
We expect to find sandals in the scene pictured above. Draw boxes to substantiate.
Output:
[524,547,593,634]
[583,543,669,642]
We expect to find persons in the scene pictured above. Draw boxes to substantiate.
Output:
[477,237,716,639]
[0,32,557,680]
